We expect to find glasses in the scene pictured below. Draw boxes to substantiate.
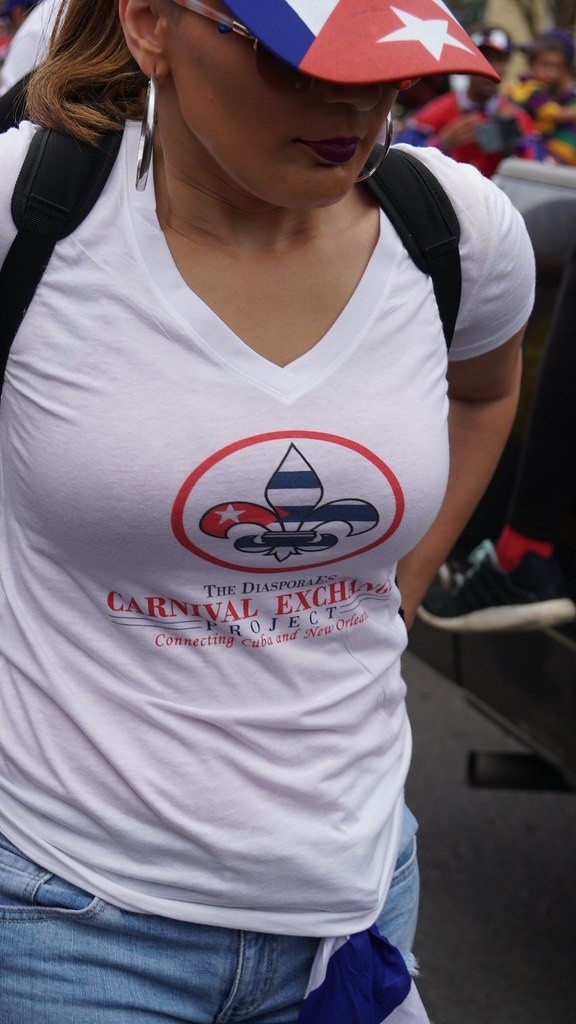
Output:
[173,0,423,97]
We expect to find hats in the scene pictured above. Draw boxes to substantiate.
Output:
[520,27,575,64]
[222,0,501,85]
[470,27,510,54]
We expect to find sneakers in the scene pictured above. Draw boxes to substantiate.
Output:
[415,539,576,631]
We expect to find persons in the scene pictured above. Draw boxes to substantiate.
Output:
[0,0,575,1023]
